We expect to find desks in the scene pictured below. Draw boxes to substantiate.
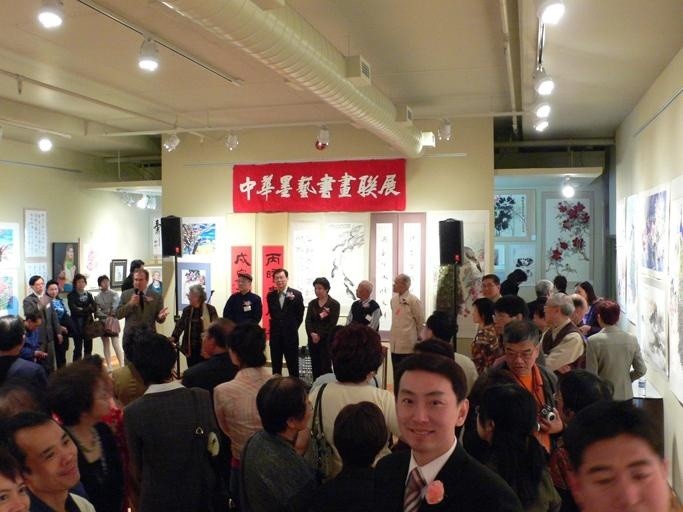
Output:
[632,375,663,400]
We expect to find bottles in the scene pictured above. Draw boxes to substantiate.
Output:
[637,374,647,399]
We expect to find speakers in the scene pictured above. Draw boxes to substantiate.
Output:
[161,216,182,257]
[438,219,464,267]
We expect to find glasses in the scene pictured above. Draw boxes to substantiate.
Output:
[503,342,537,360]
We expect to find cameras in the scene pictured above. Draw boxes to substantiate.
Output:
[538,403,556,423]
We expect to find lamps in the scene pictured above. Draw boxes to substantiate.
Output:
[562,176,574,197]
[527,1,566,133]
[136,194,156,210]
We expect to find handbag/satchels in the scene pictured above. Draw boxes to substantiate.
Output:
[104,308,121,336]
[84,306,105,340]
[191,390,229,510]
[302,381,337,484]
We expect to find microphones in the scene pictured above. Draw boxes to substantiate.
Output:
[135,287,139,295]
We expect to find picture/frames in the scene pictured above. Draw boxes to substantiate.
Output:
[541,190,594,287]
[144,264,162,294]
[112,260,127,287]
[494,189,535,241]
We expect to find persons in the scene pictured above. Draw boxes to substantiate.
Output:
[63,244,77,292]
[2,260,682,512]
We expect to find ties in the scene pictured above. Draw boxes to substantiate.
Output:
[138,291,145,312]
[402,467,427,511]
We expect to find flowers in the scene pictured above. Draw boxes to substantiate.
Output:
[426,479,445,506]
